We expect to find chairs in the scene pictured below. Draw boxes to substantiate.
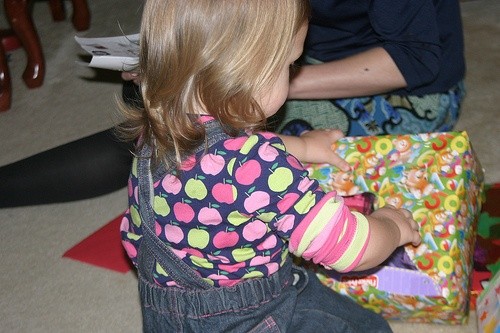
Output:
[0,0,92,112]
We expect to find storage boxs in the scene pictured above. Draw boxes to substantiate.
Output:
[298,129,484,326]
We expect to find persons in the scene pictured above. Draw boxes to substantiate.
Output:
[110,0,424,333]
[0,0,466,210]
